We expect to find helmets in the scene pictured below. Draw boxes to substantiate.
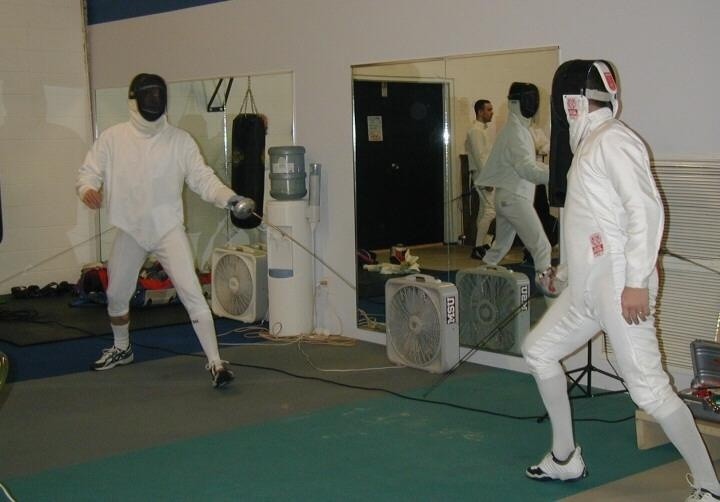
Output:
[506,82,539,127]
[126,72,168,137]
[551,58,620,156]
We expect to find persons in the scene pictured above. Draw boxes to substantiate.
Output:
[75,73,246,390]
[472,82,569,298]
[465,99,493,259]
[520,59,720,501]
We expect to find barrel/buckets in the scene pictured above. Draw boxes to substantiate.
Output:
[268,146,307,200]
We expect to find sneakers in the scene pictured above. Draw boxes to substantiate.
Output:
[205,358,234,388]
[471,244,491,260]
[683,473,720,502]
[89,343,134,371]
[525,445,589,483]
[534,266,560,298]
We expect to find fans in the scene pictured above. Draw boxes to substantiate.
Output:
[454,262,531,357]
[208,245,268,323]
[384,272,462,374]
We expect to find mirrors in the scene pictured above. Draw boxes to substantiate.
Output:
[349,44,562,364]
[93,69,296,277]
